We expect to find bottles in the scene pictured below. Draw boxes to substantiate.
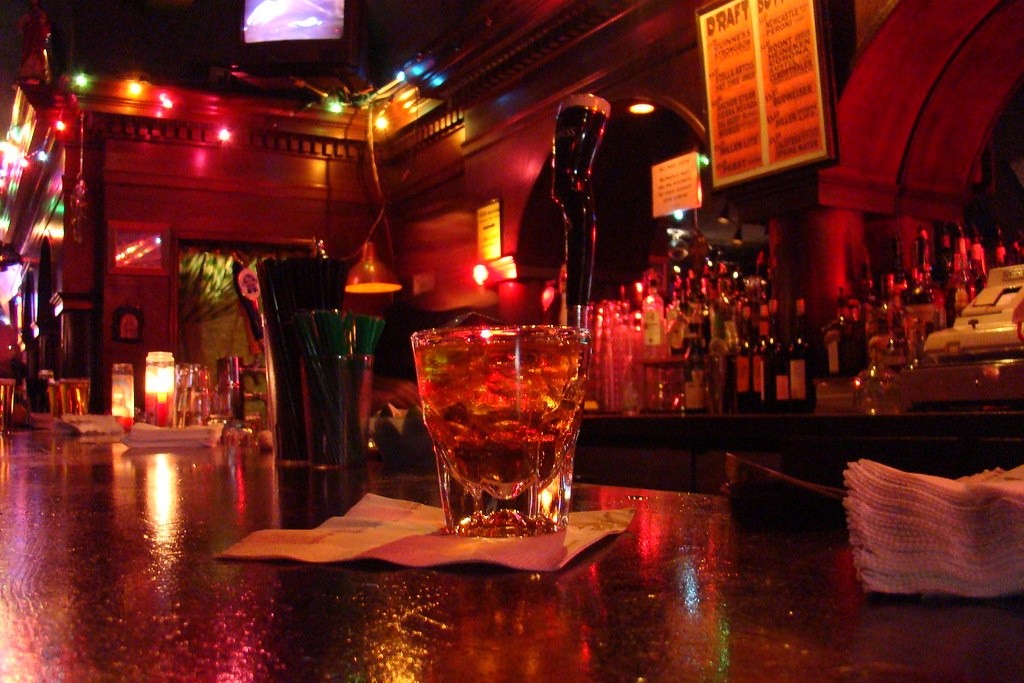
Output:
[817,221,1024,375]
[586,247,816,416]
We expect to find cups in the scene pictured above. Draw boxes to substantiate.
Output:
[410,324,592,537]
[301,353,373,470]
[0,352,260,450]
[259,305,309,467]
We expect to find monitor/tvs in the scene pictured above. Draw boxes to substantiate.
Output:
[240,0,356,61]
[34,235,49,322]
